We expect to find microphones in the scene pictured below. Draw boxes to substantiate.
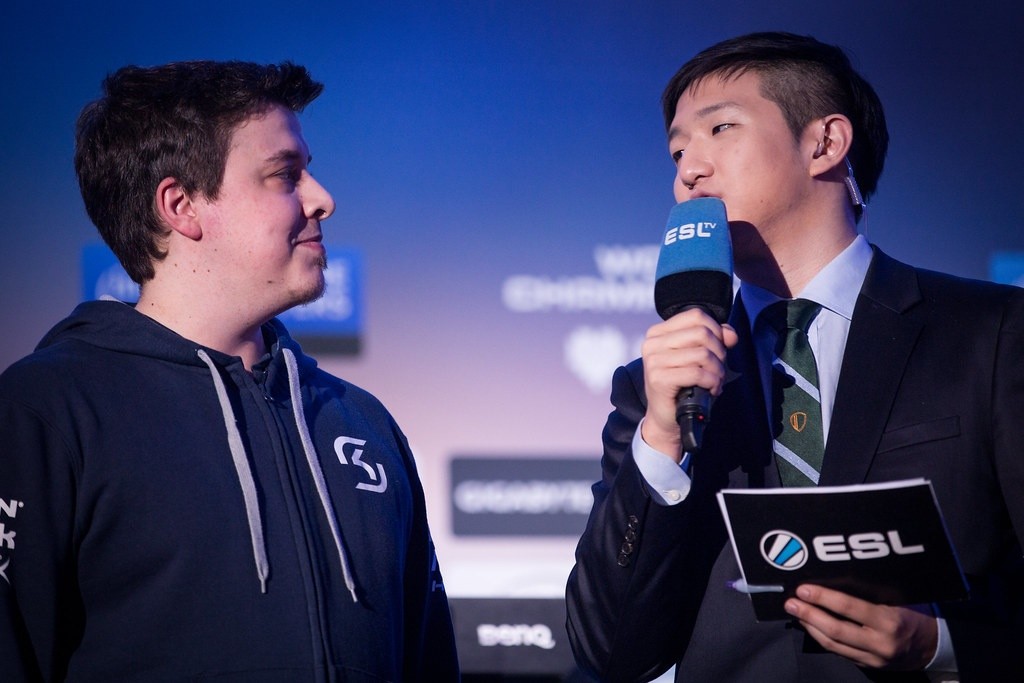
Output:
[654,197,734,453]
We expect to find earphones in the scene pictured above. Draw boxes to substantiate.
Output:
[812,141,825,158]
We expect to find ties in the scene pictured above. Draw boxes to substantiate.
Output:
[758,297,825,489]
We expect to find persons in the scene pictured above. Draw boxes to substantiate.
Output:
[563,31,1024,683]
[0,61,461,683]
